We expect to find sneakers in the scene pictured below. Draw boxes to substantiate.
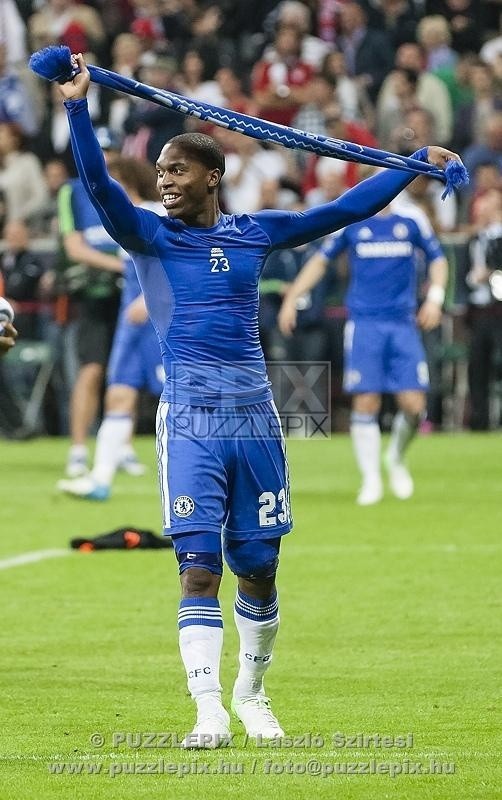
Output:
[230,699,285,740]
[180,712,230,749]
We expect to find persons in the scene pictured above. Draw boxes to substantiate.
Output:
[58,54,463,749]
